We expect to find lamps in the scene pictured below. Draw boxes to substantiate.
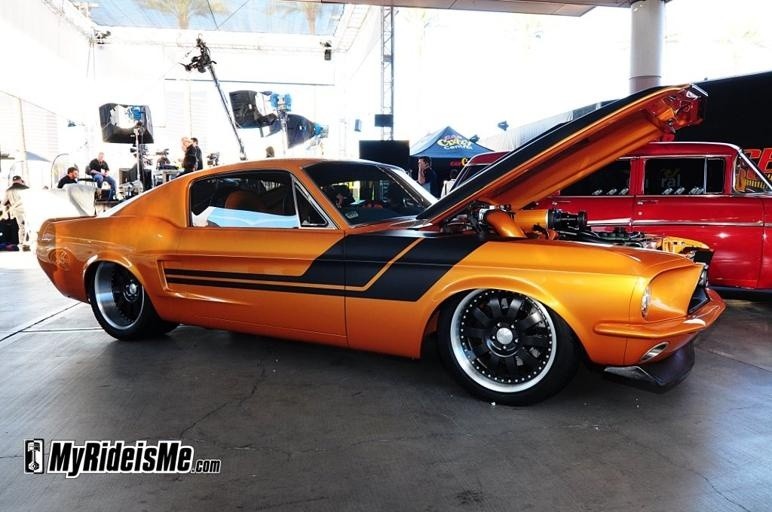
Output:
[498,120,510,131]
[319,40,332,61]
[468,134,480,143]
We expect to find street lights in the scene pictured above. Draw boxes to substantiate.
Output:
[50,152,69,189]
[7,159,25,188]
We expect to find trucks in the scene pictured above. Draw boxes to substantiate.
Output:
[498,66,771,233]
[449,140,772,315]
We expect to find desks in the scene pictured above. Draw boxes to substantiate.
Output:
[151,169,179,189]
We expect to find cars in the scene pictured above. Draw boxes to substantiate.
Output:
[35,83,727,410]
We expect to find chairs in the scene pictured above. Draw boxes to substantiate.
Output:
[225,190,271,212]
[592,186,704,196]
[85,166,97,187]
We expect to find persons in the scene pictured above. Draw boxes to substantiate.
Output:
[57,167,78,188]
[89,152,116,201]
[7,176,29,190]
[417,156,438,199]
[178,137,202,175]
[449,169,458,178]
